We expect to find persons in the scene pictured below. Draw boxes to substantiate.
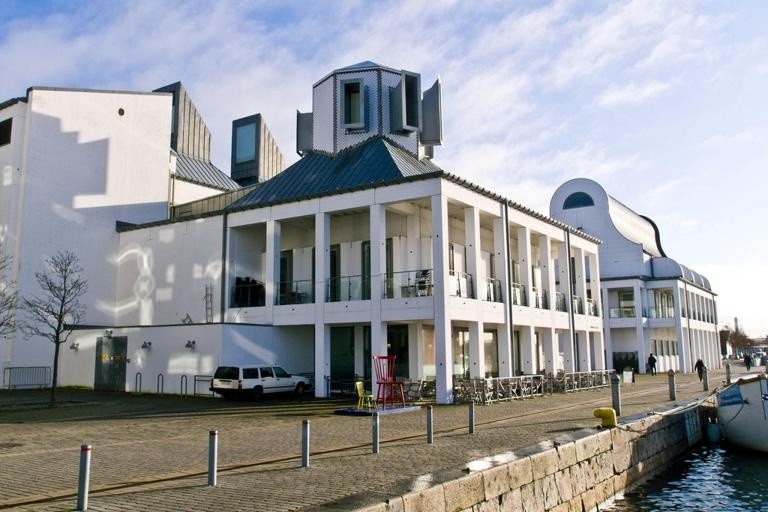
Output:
[648,352,657,376]
[744,354,751,370]
[694,359,705,381]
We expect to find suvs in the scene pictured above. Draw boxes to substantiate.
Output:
[208,365,312,402]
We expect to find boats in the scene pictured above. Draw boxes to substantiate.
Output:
[713,374,767,455]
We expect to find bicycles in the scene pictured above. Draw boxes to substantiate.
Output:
[454,383,479,406]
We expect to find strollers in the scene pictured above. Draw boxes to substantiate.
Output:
[407,378,423,403]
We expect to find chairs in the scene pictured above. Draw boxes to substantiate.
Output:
[355,355,405,409]
